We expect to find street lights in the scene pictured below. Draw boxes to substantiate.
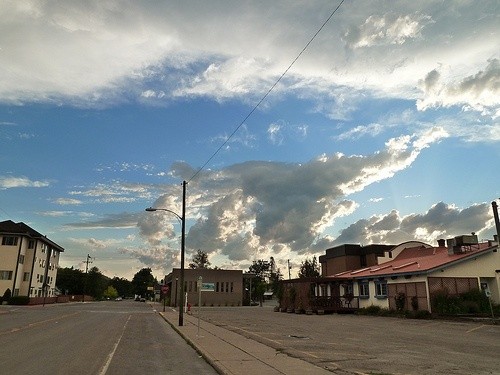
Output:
[146,180,188,326]
